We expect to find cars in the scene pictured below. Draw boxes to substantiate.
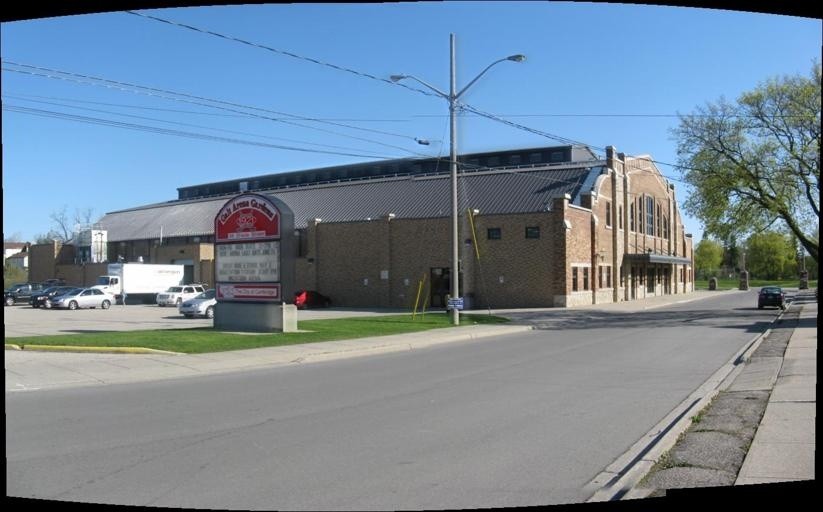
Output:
[293,290,332,310]
[1,279,116,310]
[757,287,787,310]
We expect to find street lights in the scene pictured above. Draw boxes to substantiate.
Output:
[390,54,526,326]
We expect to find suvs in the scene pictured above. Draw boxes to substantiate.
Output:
[155,283,217,317]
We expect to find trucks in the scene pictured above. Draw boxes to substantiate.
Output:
[96,262,184,305]
